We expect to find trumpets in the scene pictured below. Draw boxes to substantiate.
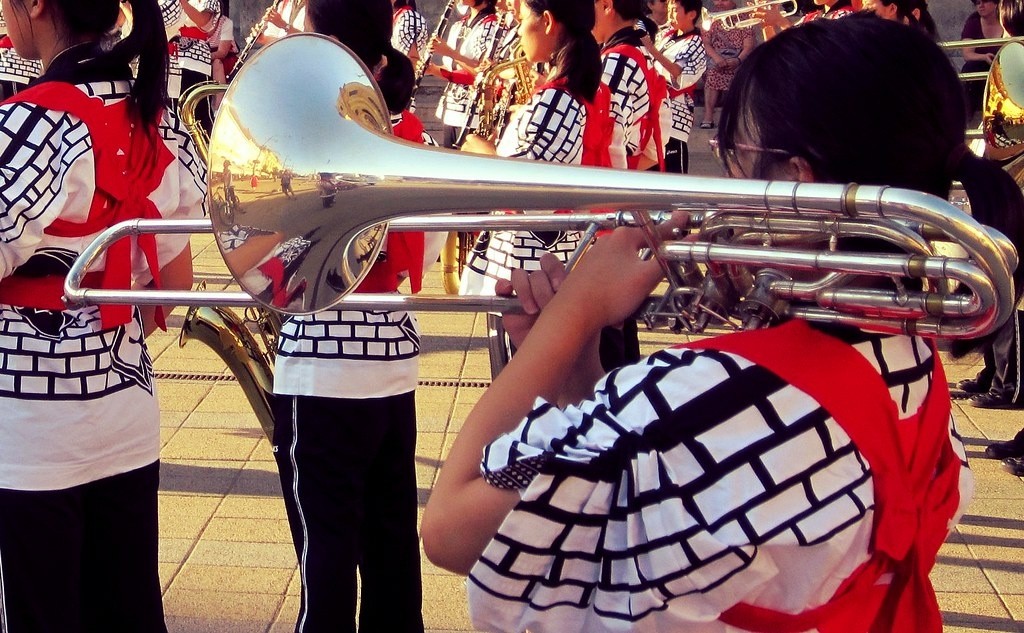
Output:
[701,0,797,31]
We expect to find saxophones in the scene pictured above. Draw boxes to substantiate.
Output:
[177,76,285,454]
[439,56,527,294]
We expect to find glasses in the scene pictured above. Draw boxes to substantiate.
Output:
[707,133,790,160]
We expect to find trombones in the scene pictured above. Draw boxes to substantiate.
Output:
[61,32,1018,342]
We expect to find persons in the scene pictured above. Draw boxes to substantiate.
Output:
[590,0,660,370]
[455,1,629,298]
[223,159,239,209]
[316,172,339,208]
[249,173,259,192]
[428,1,510,152]
[0,0,204,633]
[0,0,241,147]
[699,0,756,131]
[750,0,1024,129]
[280,167,298,201]
[422,0,1023,632]
[266,13,420,633]
[638,1,708,175]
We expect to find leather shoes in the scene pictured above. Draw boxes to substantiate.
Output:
[955,380,990,393]
[986,442,1024,460]
[968,393,1024,409]
[1002,457,1024,476]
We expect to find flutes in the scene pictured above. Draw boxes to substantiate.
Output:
[452,13,508,149]
[408,0,454,100]
[226,0,282,81]
